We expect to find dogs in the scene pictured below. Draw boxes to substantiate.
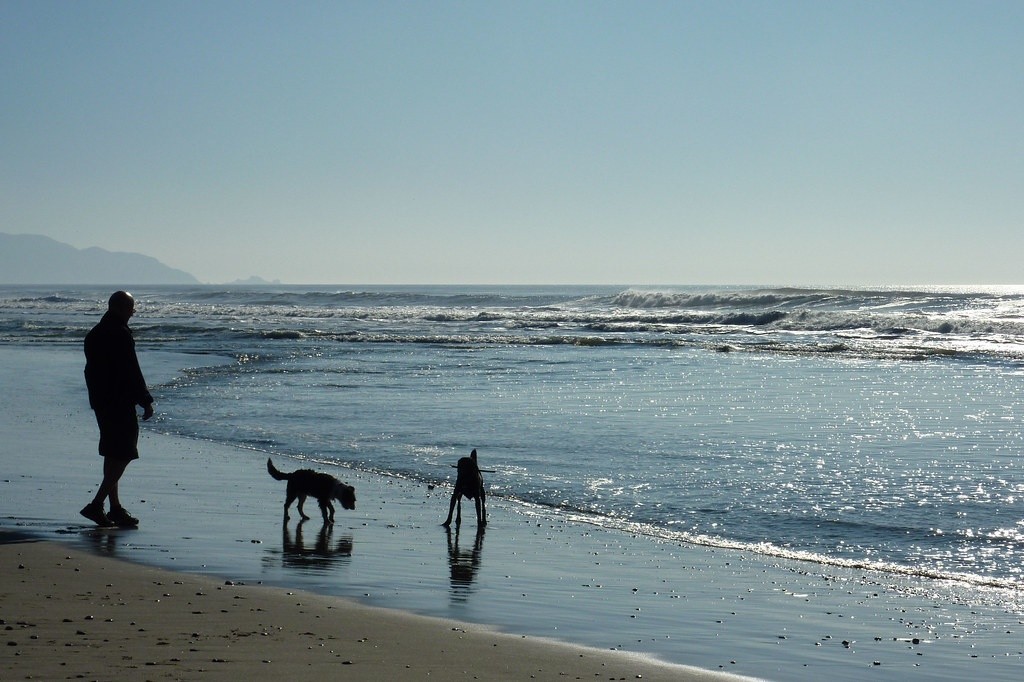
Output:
[266,457,357,525]
[439,448,488,527]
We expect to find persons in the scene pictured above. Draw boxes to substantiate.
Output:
[79,289,154,528]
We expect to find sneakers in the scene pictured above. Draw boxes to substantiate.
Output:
[80,503,115,527]
[106,508,139,527]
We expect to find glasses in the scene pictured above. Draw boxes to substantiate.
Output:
[125,306,136,313]
